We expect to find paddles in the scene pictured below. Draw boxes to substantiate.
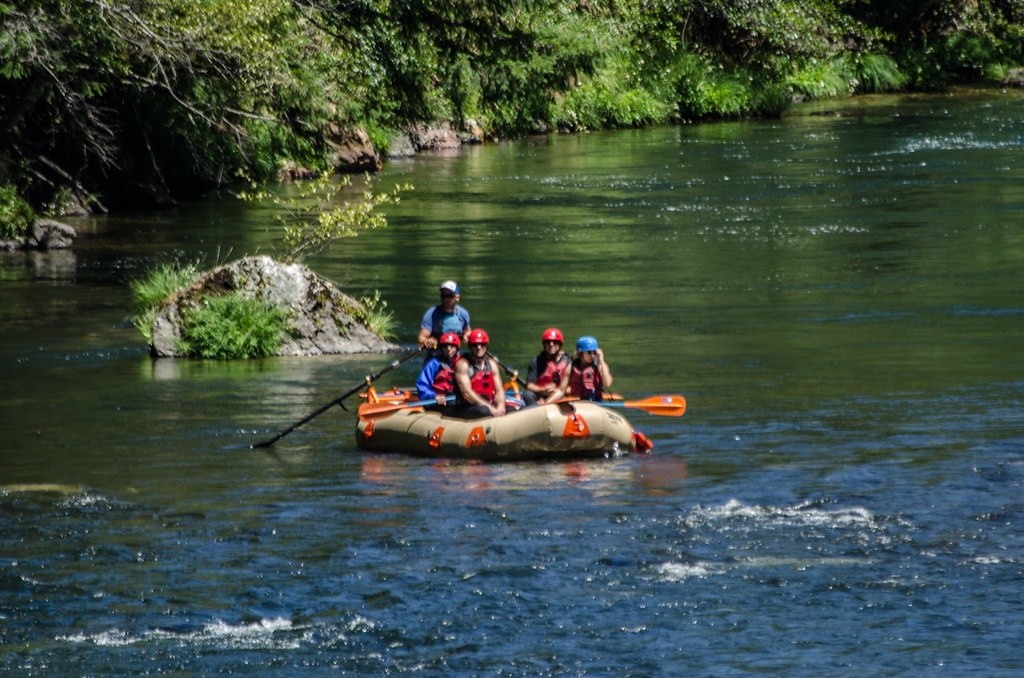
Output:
[532,385,691,419]
[463,324,530,390]
[357,385,544,421]
[252,341,430,449]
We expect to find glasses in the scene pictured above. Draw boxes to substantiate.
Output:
[587,350,596,355]
[441,294,456,299]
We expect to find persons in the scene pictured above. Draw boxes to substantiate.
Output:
[523,328,572,404]
[416,330,464,417]
[454,328,517,424]
[418,281,472,367]
[540,336,612,407]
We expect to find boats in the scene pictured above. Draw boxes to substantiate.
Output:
[354,377,656,461]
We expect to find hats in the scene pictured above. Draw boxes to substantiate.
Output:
[440,279,460,296]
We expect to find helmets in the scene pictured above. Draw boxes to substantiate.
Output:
[439,333,461,349]
[468,328,489,344]
[542,328,563,344]
[576,335,598,352]
